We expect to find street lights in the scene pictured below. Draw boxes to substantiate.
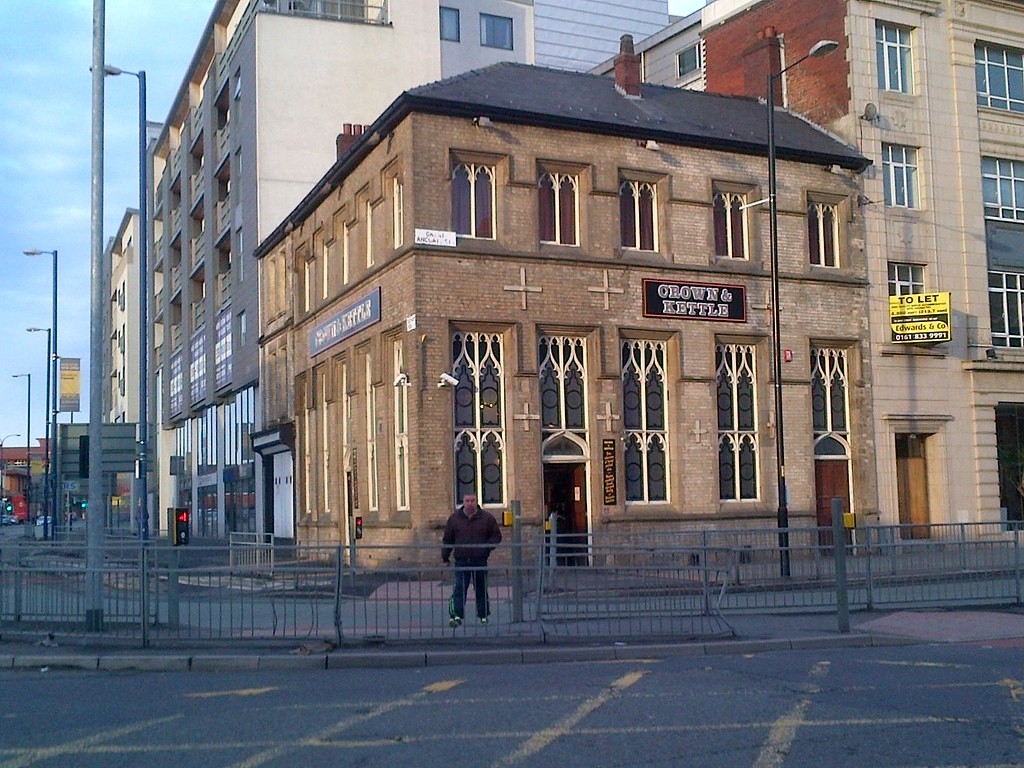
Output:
[92,62,154,551]
[12,373,33,524]
[763,39,840,581]
[0,435,20,486]
[25,249,62,547]
[27,326,49,538]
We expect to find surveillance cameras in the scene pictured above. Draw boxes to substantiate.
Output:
[440,373,459,386]
[394,374,405,386]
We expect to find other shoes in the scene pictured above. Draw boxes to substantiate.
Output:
[449,618,458,627]
[481,617,488,624]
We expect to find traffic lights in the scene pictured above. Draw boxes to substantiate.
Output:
[81,502,86,514]
[5,502,11,514]
[352,514,365,543]
[170,505,192,548]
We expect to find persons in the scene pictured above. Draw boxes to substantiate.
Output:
[441,491,501,627]
